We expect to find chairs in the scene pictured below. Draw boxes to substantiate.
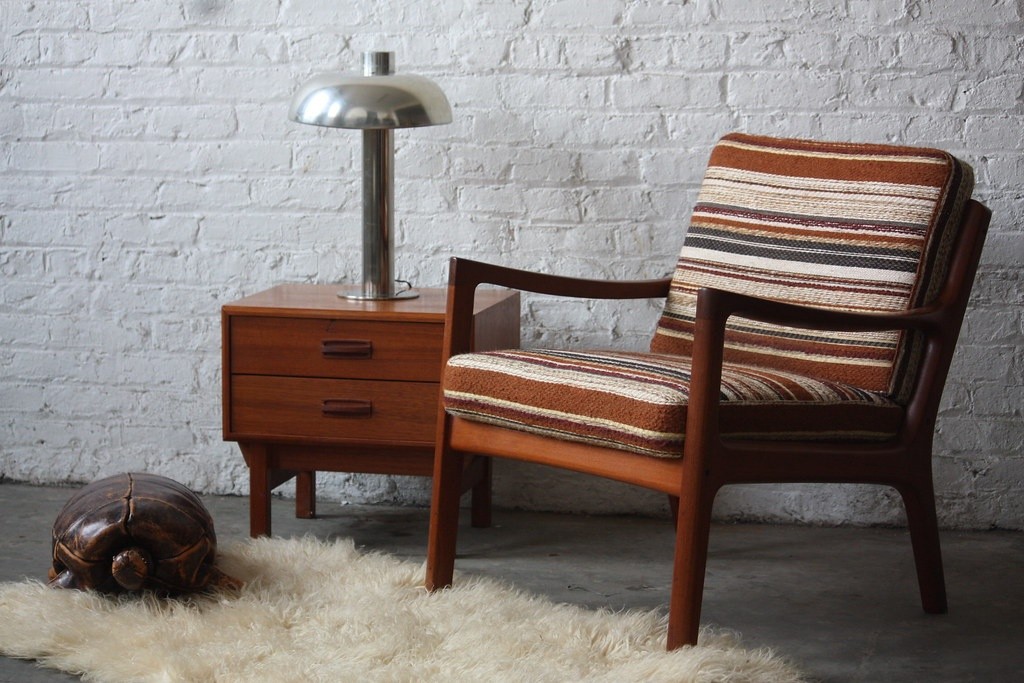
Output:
[425,134,994,652]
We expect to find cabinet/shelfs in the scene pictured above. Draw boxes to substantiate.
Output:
[220,281,520,543]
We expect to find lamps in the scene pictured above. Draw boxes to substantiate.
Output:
[288,49,454,301]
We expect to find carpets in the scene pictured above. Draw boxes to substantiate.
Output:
[0,530,805,683]
